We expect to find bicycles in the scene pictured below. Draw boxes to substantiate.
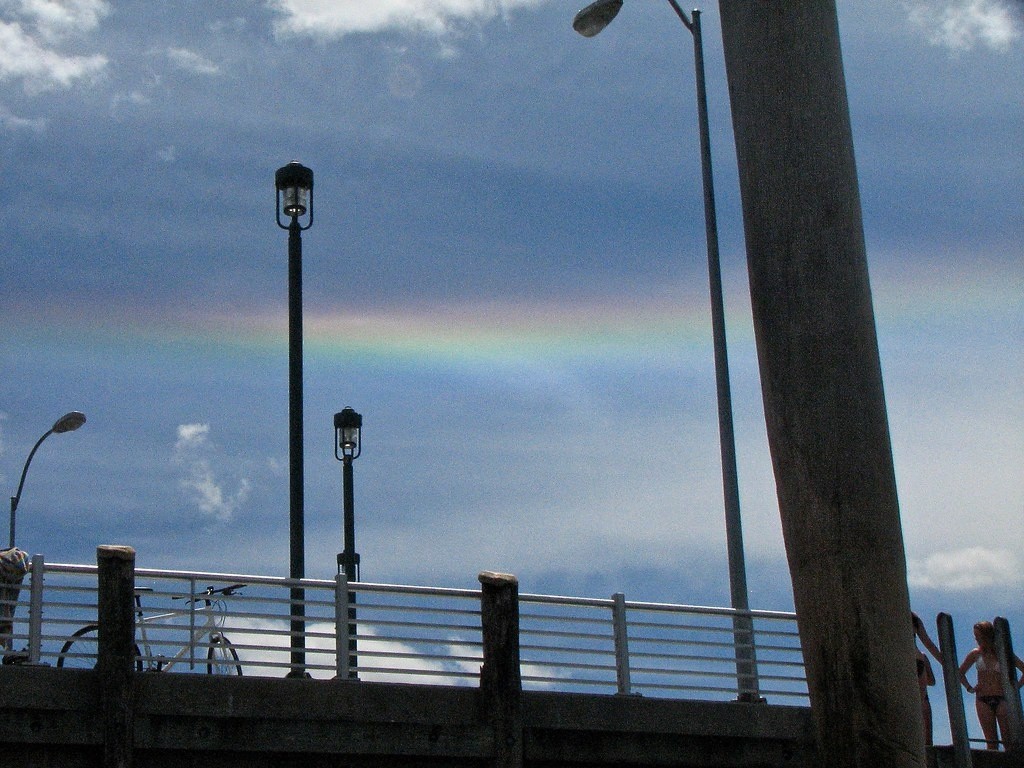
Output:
[57,584,246,675]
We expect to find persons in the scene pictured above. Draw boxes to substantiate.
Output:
[911,609,942,746]
[960,619,1024,752]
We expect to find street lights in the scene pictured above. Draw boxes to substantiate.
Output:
[273,160,313,678]
[9,410,89,551]
[575,0,765,703]
[332,404,363,680]
